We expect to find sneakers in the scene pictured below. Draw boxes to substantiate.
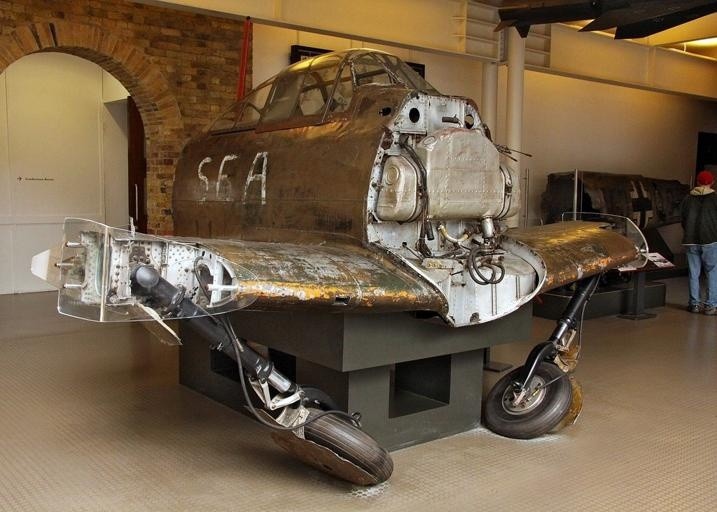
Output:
[687,305,717,315]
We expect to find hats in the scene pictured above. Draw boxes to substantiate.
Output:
[697,171,715,185]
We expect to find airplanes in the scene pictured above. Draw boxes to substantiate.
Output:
[29,47,649,485]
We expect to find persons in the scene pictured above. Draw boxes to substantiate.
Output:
[678,170,716,315]
